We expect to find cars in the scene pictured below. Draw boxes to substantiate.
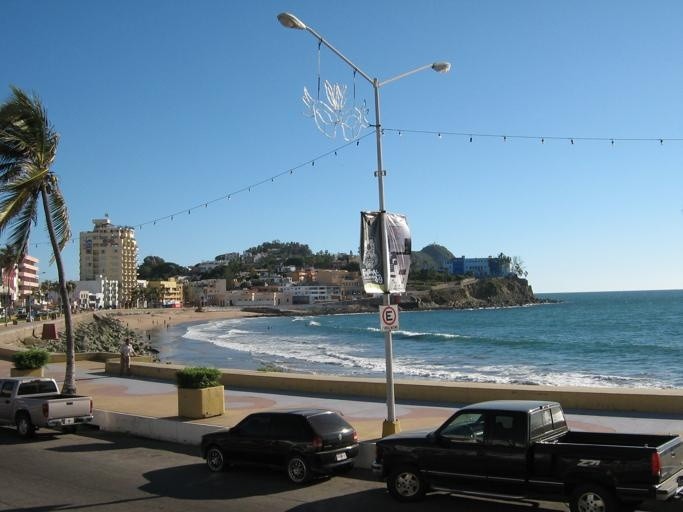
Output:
[202,409,359,484]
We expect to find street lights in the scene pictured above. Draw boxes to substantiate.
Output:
[277,11,450,437]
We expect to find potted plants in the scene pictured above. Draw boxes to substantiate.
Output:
[174,364,226,420]
[8,347,52,377]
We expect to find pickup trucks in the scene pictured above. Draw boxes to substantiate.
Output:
[0,378,94,437]
[372,400,672,512]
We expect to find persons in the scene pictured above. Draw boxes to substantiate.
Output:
[118,339,138,377]
